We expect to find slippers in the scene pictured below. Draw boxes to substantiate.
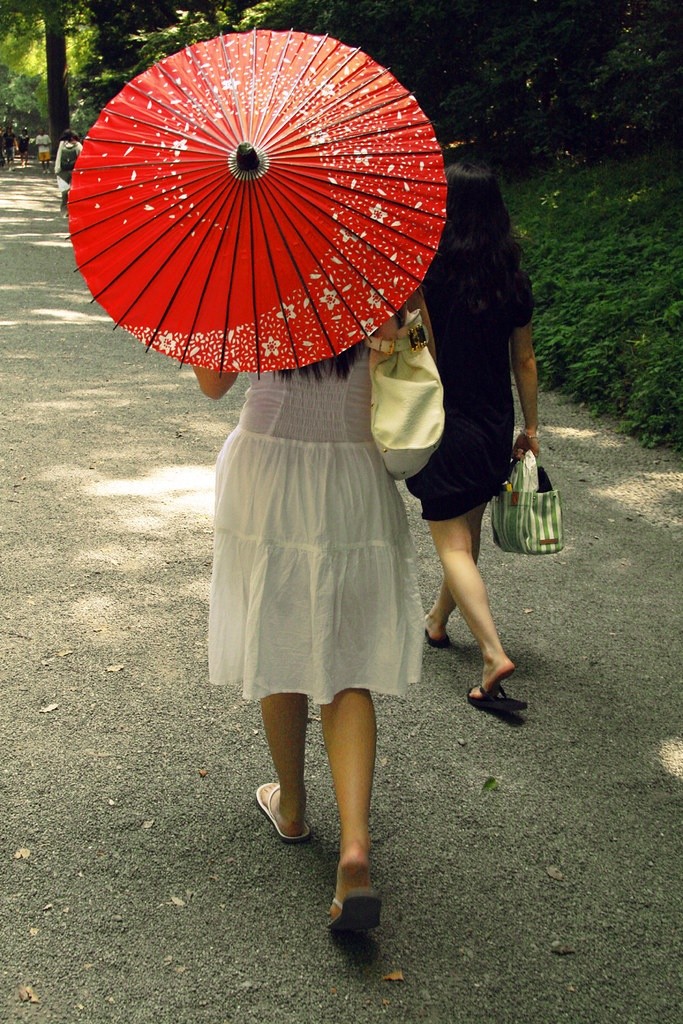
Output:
[425,629,450,648]
[326,890,381,930]
[256,783,311,844]
[467,684,527,711]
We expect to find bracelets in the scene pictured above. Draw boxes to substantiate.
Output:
[522,433,537,438]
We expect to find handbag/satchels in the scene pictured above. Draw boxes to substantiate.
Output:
[364,299,445,480]
[491,450,563,555]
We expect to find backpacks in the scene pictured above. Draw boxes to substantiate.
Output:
[60,141,77,171]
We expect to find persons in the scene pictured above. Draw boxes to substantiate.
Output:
[35,129,52,174]
[422,160,540,710]
[0,130,5,167]
[17,129,30,166]
[3,126,18,171]
[54,129,83,218]
[192,287,438,930]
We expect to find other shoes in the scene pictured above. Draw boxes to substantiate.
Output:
[43,170,46,174]
[47,169,51,174]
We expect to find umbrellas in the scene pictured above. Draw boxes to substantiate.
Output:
[66,27,449,380]
